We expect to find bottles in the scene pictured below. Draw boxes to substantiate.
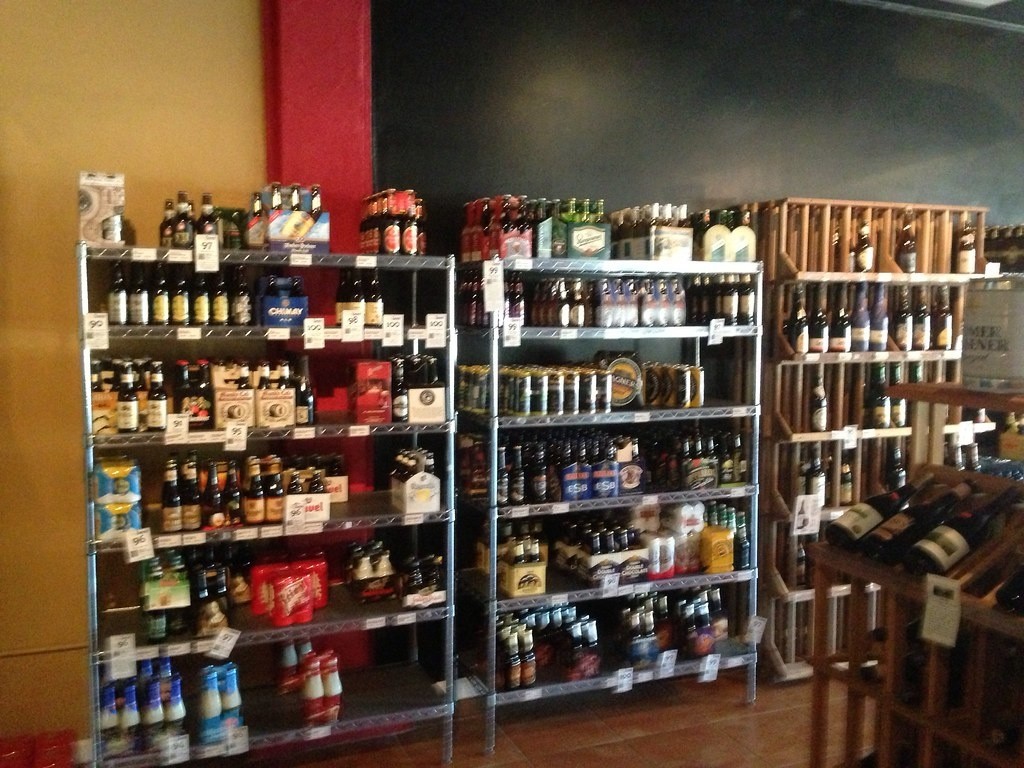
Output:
[457,265,758,329]
[100,634,344,759]
[91,352,316,434]
[848,219,874,272]
[390,349,440,422]
[161,447,325,533]
[795,406,1024,747]
[900,213,916,272]
[958,219,976,273]
[106,261,385,327]
[461,194,754,263]
[789,281,955,355]
[138,538,284,646]
[984,224,1024,239]
[105,181,426,257]
[485,501,749,691]
[389,444,435,514]
[342,537,447,608]
[809,362,927,431]
[464,419,745,507]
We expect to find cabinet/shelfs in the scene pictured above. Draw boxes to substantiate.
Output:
[742,197,992,688]
[877,374,1023,420]
[797,454,1024,768]
[73,237,461,765]
[453,251,768,757]
